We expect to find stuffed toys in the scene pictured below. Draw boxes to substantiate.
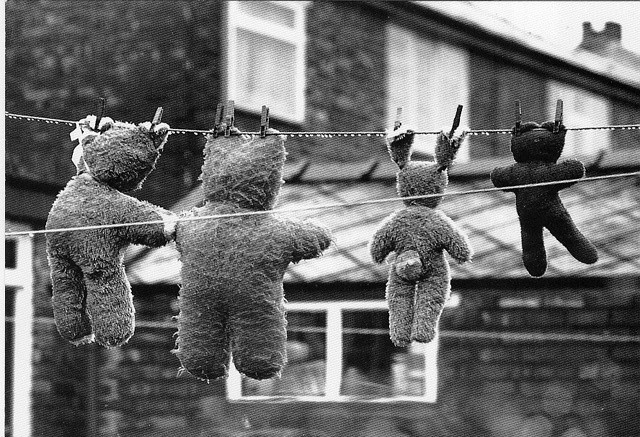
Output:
[372,130,472,347]
[178,128,333,381]
[45,117,177,345]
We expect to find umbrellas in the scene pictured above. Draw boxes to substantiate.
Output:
[492,121,598,276]
